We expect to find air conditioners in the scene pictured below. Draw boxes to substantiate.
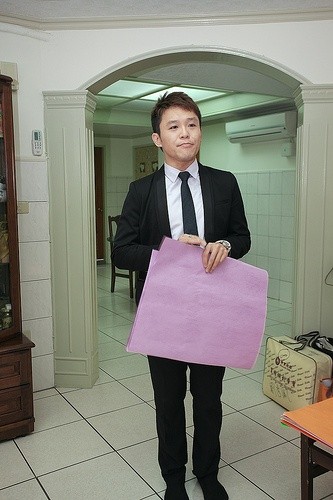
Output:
[223,112,298,144]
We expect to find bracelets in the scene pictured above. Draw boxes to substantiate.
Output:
[218,241,230,250]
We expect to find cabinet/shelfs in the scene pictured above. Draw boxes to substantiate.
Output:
[0,73,36,443]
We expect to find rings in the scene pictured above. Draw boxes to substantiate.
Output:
[189,235,192,238]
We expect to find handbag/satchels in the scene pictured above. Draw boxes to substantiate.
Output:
[293,331,333,360]
[262,335,332,412]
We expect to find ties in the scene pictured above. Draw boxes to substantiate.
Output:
[178,171,200,247]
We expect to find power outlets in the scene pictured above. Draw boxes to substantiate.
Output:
[23,330,31,341]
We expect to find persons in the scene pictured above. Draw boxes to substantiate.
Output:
[111,92,252,500]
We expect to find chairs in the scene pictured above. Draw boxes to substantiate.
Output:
[107,215,135,299]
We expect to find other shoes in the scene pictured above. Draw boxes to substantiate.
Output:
[164,485,189,500]
[195,473,229,500]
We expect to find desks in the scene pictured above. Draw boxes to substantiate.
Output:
[280,398,333,500]
